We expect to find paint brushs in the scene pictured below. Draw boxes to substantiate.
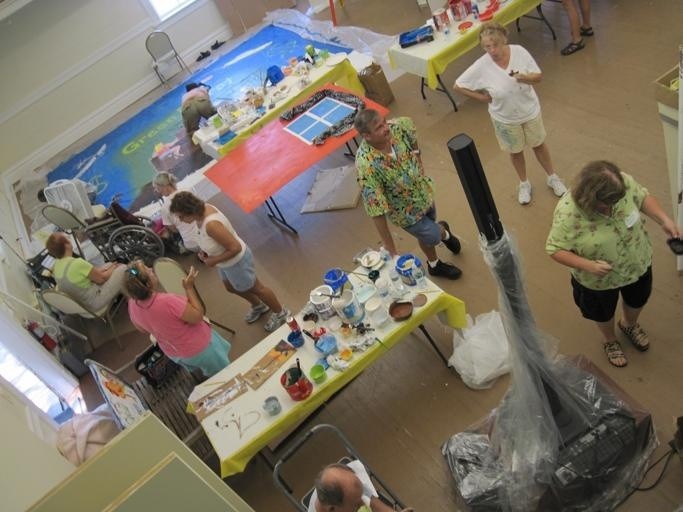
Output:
[296,359,301,377]
[302,330,317,341]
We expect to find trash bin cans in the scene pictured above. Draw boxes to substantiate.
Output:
[653,65,679,219]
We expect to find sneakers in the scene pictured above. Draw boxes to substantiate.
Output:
[243,301,270,324]
[547,174,567,196]
[438,221,461,254]
[264,305,292,331]
[518,180,533,205]
[427,259,462,280]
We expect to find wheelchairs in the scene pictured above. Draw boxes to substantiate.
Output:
[81,192,165,274]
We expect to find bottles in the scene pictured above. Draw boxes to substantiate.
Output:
[394,254,427,292]
[380,246,403,291]
[442,22,450,38]
[323,266,353,292]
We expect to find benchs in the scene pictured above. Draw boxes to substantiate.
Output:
[80,337,257,468]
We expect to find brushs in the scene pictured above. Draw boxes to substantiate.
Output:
[310,290,339,298]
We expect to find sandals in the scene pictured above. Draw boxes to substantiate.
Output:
[617,319,650,352]
[578,25,595,36]
[560,36,586,56]
[603,340,628,367]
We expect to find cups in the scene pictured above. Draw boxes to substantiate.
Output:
[278,368,313,400]
[309,363,327,383]
[432,0,467,31]
[261,396,280,415]
[309,284,365,361]
[287,332,304,347]
[305,45,315,57]
[211,116,222,128]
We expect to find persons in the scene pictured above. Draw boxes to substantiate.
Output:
[307,463,414,512]
[354,109,462,280]
[545,160,683,368]
[46,232,130,313]
[560,0,594,55]
[169,191,292,332]
[151,172,206,256]
[181,83,217,136]
[119,259,231,378]
[453,22,567,205]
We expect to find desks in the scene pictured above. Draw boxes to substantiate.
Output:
[201,81,391,234]
[385,1,555,114]
[183,249,468,497]
[190,48,366,165]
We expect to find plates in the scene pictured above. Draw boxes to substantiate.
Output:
[457,22,473,31]
[356,283,383,313]
[326,53,346,66]
[666,237,682,256]
[360,252,380,267]
[387,299,412,320]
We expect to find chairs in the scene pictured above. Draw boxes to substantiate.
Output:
[270,423,410,511]
[40,266,141,353]
[39,202,107,262]
[144,30,191,90]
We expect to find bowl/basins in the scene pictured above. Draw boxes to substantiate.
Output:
[262,65,283,93]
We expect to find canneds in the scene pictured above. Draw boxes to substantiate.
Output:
[286,315,300,332]
